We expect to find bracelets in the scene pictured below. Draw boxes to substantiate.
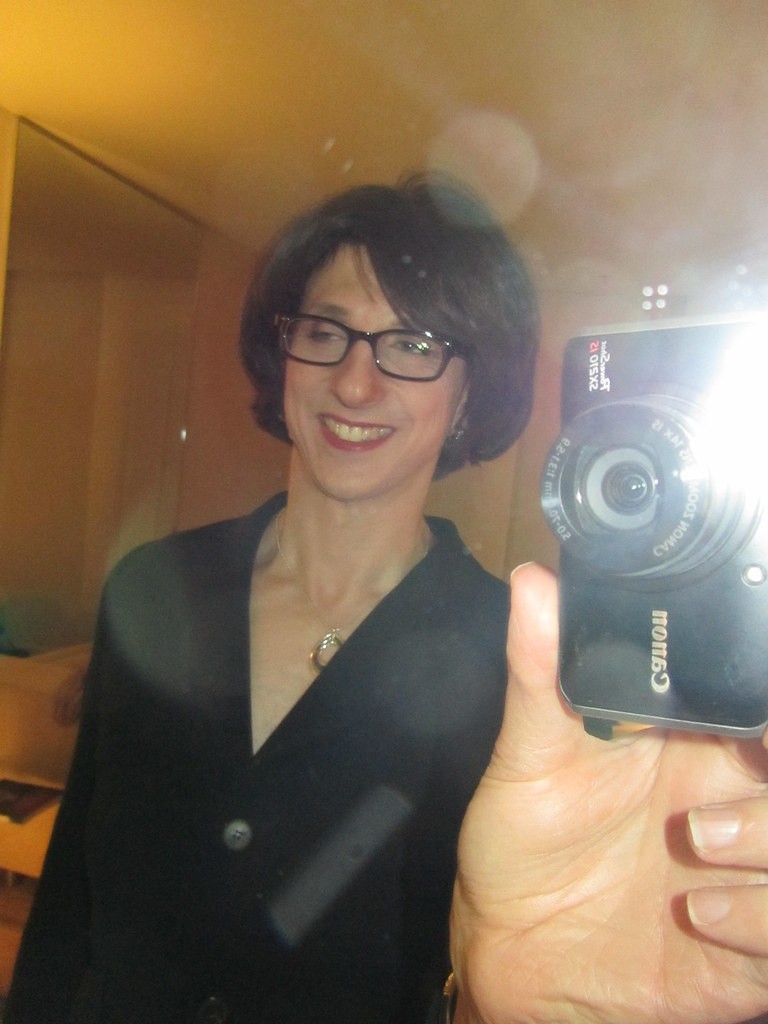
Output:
[438,973,458,1024]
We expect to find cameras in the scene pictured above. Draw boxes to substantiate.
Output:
[539,307,768,740]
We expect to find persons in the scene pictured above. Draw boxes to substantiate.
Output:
[0,168,768,1024]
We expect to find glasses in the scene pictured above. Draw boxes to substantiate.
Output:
[271,310,473,381]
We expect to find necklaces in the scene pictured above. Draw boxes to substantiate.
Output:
[275,507,431,675]
